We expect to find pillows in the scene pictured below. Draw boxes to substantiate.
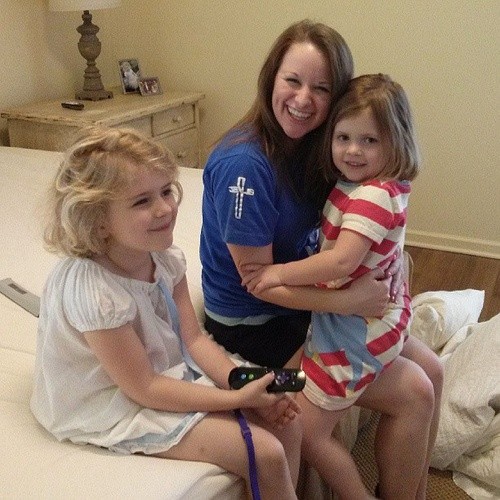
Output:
[407,288,485,351]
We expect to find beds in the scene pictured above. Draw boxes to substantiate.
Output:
[0,146,415,500]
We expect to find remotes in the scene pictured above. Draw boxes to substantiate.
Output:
[61,101,84,109]
[0,278,41,317]
[228,366,306,392]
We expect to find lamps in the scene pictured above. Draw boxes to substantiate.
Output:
[48,0,122,101]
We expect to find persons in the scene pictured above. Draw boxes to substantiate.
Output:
[30,128,302,500]
[199,17,444,500]
[122,63,159,94]
[242,73,419,500]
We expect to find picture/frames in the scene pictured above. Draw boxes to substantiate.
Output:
[116,58,143,95]
[138,77,163,97]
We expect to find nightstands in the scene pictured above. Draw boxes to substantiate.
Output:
[0,86,206,169]
